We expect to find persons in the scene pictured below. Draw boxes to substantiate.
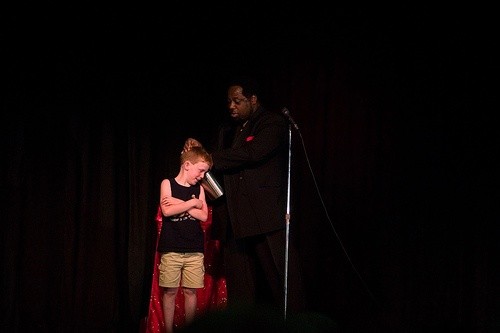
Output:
[158,146,214,333]
[182,80,305,314]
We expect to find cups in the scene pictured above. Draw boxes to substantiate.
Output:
[198,171,224,199]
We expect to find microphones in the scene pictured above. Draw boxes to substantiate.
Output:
[282,108,299,130]
